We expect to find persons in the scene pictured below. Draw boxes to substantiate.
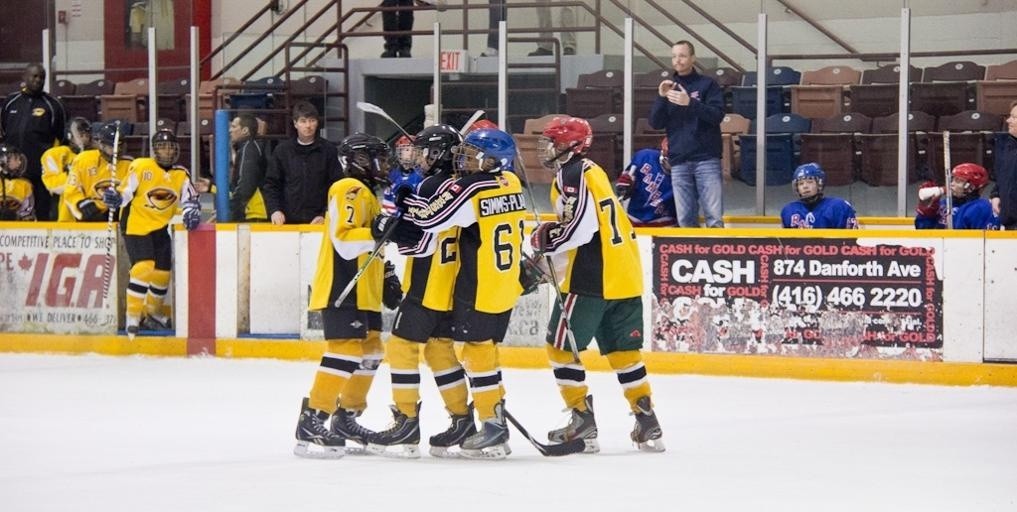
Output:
[204,101,345,224]
[381,0,415,57]
[779,161,861,228]
[102,128,201,336]
[527,0,578,56]
[0,139,38,223]
[913,160,1000,233]
[0,64,65,219]
[611,137,669,226]
[482,0,507,56]
[39,115,93,215]
[991,101,1017,231]
[529,115,663,445]
[294,120,526,458]
[646,40,727,229]
[65,121,137,222]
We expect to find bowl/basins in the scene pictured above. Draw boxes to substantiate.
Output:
[145,187,177,212]
[94,178,122,200]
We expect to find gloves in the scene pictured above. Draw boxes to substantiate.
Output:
[531,221,559,256]
[381,260,403,309]
[371,213,423,247]
[75,197,100,218]
[103,186,124,207]
[379,182,417,219]
[514,256,550,295]
[915,178,945,219]
[179,189,202,231]
[614,172,633,200]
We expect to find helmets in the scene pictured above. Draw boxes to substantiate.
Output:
[412,123,464,177]
[62,115,93,149]
[393,134,416,171]
[95,120,123,164]
[336,130,392,180]
[466,120,498,130]
[456,126,519,178]
[491,153,498,157]
[149,127,179,168]
[950,162,989,199]
[790,161,826,201]
[543,113,592,158]
[0,142,27,178]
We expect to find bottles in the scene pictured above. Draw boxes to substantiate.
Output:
[918,186,946,201]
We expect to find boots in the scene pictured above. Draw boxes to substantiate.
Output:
[459,398,510,450]
[295,395,347,447]
[546,393,601,440]
[428,400,478,450]
[364,398,424,446]
[327,398,380,444]
[629,394,663,444]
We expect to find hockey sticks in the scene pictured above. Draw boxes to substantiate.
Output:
[503,409,586,456]
[100,120,121,327]
[354,102,557,288]
[334,108,485,308]
